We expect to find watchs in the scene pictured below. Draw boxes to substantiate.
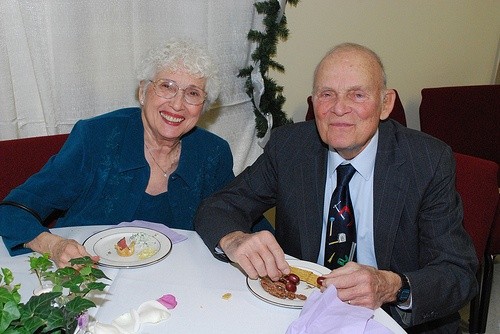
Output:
[388,270,411,307]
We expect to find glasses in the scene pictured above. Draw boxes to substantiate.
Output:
[149,79,209,105]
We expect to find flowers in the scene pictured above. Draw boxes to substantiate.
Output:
[0,251,112,334]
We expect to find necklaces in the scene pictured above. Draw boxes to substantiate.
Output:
[142,141,182,178]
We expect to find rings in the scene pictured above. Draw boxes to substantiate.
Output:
[348,300,350,304]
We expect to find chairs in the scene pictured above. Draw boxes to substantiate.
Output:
[411,152,500,334]
[419,83,500,169]
[0,132,69,206]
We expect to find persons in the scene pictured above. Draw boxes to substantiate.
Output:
[193,42,481,334]
[0,40,275,280]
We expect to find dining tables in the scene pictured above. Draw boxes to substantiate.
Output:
[0,225,408,334]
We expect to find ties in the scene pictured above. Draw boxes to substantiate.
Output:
[324,163,358,273]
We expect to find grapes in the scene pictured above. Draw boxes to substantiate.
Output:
[317,276,326,285]
[280,273,299,292]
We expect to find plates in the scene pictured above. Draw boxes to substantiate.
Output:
[245,259,333,310]
[81,226,173,269]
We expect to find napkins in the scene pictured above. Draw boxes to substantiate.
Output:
[117,220,187,244]
[285,284,394,333]
